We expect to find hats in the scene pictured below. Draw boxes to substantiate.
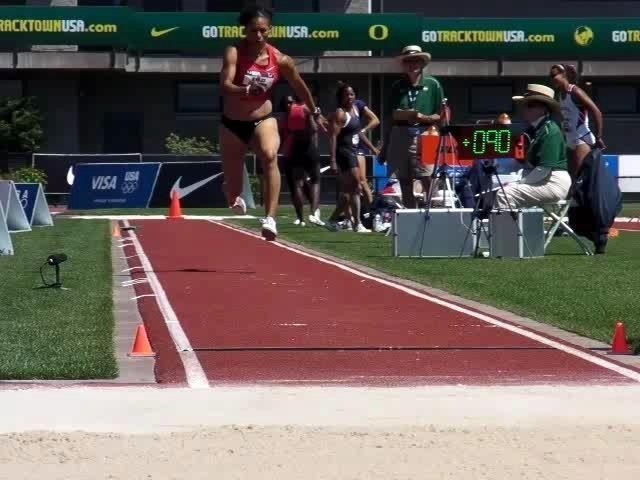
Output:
[512,83,558,106]
[394,45,432,64]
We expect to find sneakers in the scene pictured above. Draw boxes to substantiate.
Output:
[259,217,277,241]
[230,196,247,214]
[293,208,392,233]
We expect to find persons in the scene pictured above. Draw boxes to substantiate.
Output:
[546,61,608,182]
[218,4,330,244]
[282,83,326,228]
[325,78,382,233]
[388,44,449,208]
[496,111,514,127]
[277,89,313,205]
[488,82,575,211]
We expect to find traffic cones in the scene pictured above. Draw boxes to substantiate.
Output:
[166,188,186,221]
[606,323,634,355]
[130,326,156,359]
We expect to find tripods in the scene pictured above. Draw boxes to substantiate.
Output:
[460,164,535,260]
[418,99,490,258]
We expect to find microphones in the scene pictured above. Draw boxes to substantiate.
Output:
[47,253,68,266]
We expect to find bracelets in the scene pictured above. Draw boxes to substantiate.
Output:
[238,83,250,97]
[309,106,322,122]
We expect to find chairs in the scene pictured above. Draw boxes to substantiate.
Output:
[538,148,595,258]
